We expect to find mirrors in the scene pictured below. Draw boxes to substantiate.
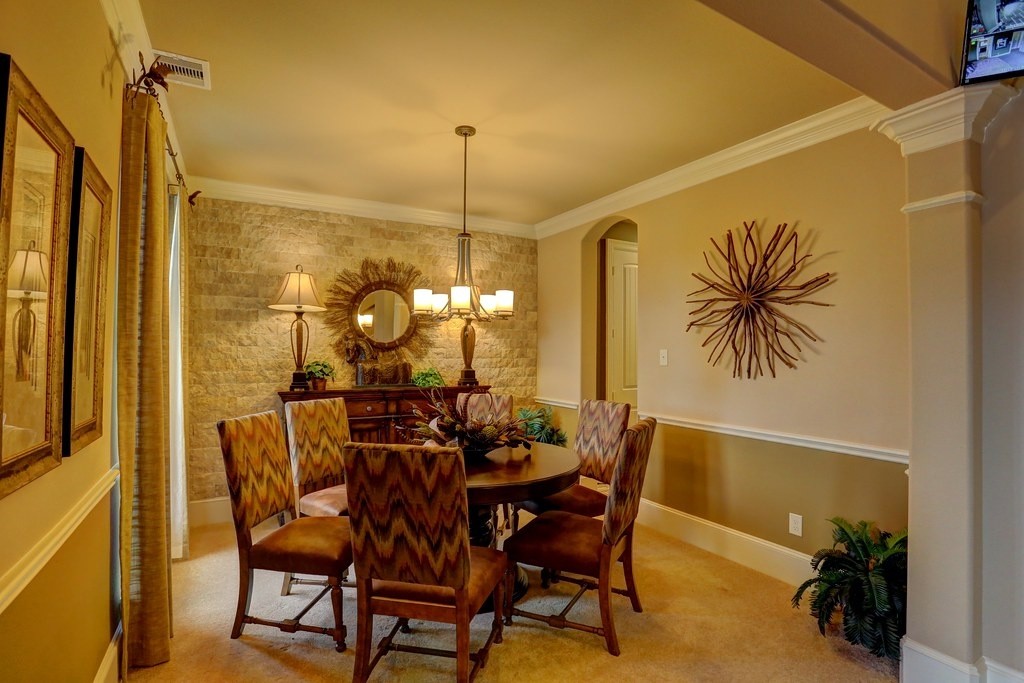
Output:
[348,281,417,352]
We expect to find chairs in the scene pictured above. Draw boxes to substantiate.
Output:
[342,442,509,683]
[280,397,356,597]
[510,398,632,583]
[503,417,657,658]
[216,410,353,653]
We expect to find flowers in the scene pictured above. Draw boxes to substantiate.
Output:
[392,386,536,452]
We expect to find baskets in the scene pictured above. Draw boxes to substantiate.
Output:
[439,388,505,466]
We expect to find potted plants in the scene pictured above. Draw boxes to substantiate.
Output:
[304,360,337,391]
[790,517,908,667]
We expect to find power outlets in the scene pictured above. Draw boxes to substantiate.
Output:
[788,512,803,538]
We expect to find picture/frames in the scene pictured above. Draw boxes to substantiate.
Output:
[62,146,113,458]
[0,53,63,500]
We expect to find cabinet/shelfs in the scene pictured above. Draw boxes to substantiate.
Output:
[278,385,492,444]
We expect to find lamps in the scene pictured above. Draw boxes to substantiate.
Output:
[267,264,327,391]
[451,285,491,386]
[410,134,516,323]
[6,237,50,381]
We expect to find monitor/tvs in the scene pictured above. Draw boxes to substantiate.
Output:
[959,0,1024,86]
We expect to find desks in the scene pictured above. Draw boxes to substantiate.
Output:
[423,439,582,614]
